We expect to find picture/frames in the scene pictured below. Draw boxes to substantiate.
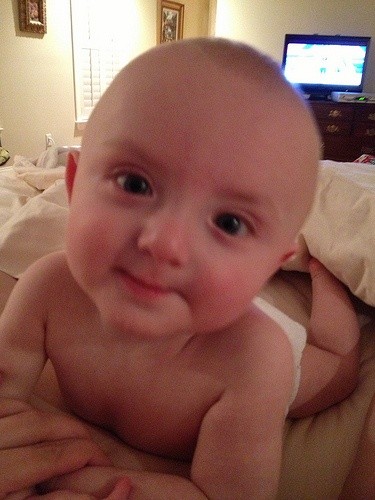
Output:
[157,0,185,47]
[19,0,47,35]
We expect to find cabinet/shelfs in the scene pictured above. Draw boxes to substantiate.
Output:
[306,99,375,164]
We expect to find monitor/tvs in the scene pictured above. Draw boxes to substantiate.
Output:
[281,34,371,101]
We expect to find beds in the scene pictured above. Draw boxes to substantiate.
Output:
[0,146,374,500]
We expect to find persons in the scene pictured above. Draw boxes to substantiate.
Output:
[0,398,132,499]
[0,38,360,500]
